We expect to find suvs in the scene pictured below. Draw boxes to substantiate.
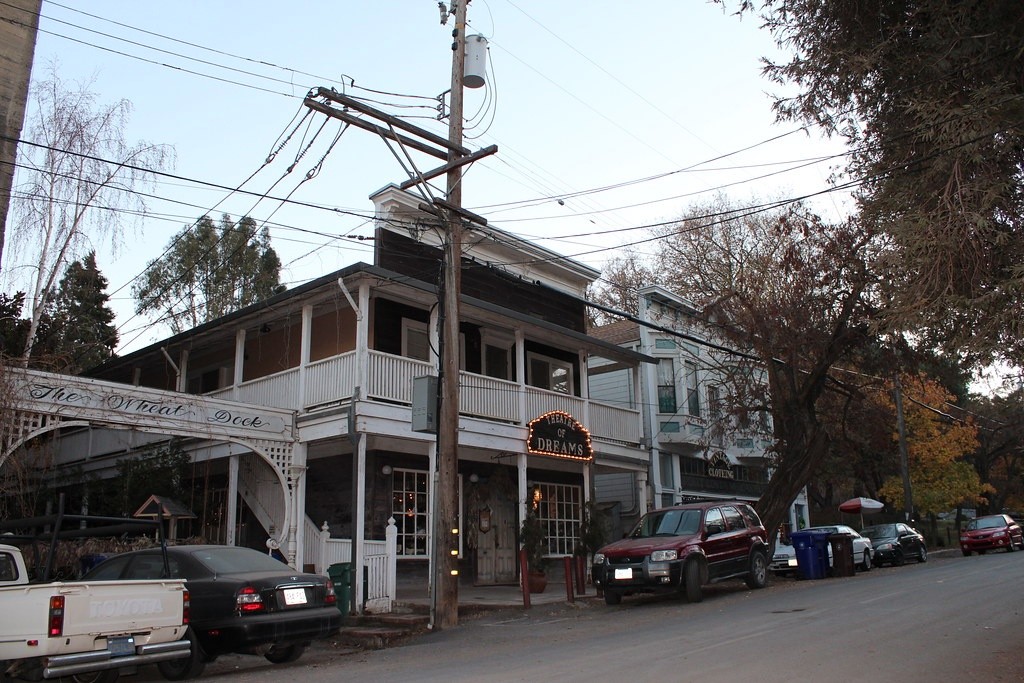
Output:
[592,501,772,604]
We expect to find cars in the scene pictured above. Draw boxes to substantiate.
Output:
[858,522,927,567]
[765,525,875,577]
[72,545,344,680]
[959,514,1024,556]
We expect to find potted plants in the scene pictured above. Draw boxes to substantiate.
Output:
[520,518,547,594]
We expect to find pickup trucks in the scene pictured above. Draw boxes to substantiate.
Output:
[0,544,192,683]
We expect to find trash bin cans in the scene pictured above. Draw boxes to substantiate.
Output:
[326,562,368,615]
[791,527,857,579]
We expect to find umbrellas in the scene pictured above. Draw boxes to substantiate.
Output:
[838,497,884,530]
